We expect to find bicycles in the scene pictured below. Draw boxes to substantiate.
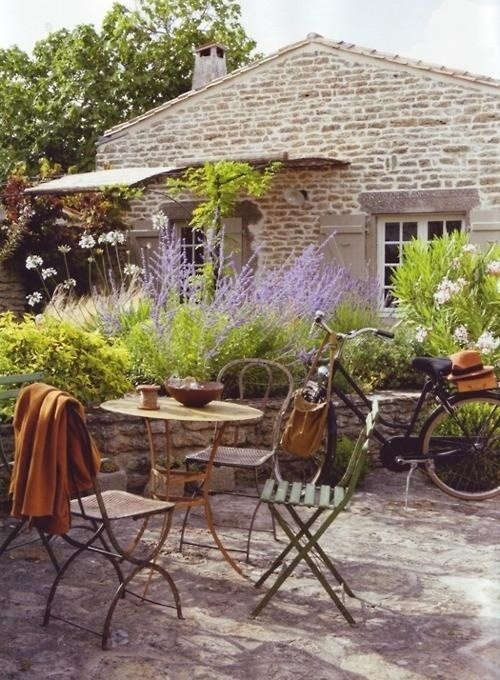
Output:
[273,305,499,501]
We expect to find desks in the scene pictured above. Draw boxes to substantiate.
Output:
[97,394,265,603]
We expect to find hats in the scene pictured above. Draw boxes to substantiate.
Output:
[448,350,494,379]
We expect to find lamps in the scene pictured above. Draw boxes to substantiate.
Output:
[283,188,307,207]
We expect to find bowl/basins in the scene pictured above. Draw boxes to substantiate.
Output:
[168,380,225,407]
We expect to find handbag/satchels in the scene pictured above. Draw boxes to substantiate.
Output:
[280,388,329,459]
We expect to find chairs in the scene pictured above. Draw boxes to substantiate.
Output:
[0,385,184,650]
[249,397,381,627]
[180,358,294,556]
